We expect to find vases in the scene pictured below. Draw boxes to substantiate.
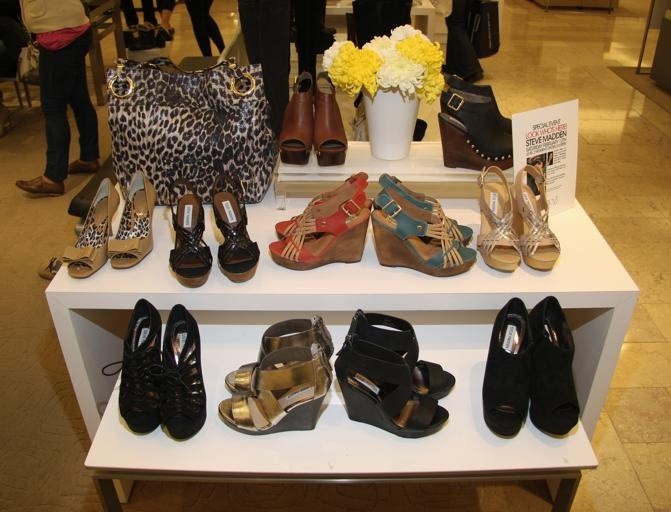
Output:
[363,88,421,160]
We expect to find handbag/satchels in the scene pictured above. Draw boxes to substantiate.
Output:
[105,56,279,206]
[469,2,499,58]
[18,41,40,86]
[124,22,165,51]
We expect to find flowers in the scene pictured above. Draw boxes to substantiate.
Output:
[321,23,447,104]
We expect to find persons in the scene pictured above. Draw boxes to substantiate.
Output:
[120,1,156,31]
[237,1,327,143]
[156,1,175,37]
[183,1,226,57]
[19,0,101,195]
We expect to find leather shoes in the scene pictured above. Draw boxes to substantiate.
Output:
[16,176,64,197]
[68,159,100,174]
[442,64,483,83]
[160,27,174,40]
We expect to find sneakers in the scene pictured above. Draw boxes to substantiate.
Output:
[107,171,157,269]
[39,257,62,280]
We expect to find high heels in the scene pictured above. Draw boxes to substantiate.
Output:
[440,74,512,135]
[372,174,473,246]
[62,177,120,278]
[529,296,580,439]
[225,315,334,401]
[335,333,449,438]
[483,297,534,439]
[218,345,332,435]
[164,179,212,288]
[510,165,560,271]
[278,72,313,164]
[477,166,522,272]
[148,304,206,441]
[275,172,372,240]
[371,209,476,276]
[345,309,455,400]
[102,299,162,435]
[209,173,260,283]
[269,190,371,270]
[312,72,348,166]
[438,88,513,171]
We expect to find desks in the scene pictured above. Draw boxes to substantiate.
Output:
[0,1,129,109]
[83,311,598,512]
[44,139,641,446]
[323,0,435,41]
